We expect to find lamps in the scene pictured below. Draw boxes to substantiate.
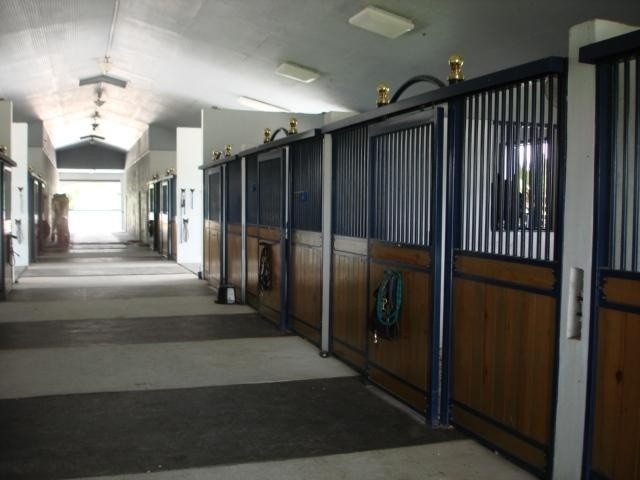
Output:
[88,55,114,145]
[274,62,321,84]
[348,6,415,40]
